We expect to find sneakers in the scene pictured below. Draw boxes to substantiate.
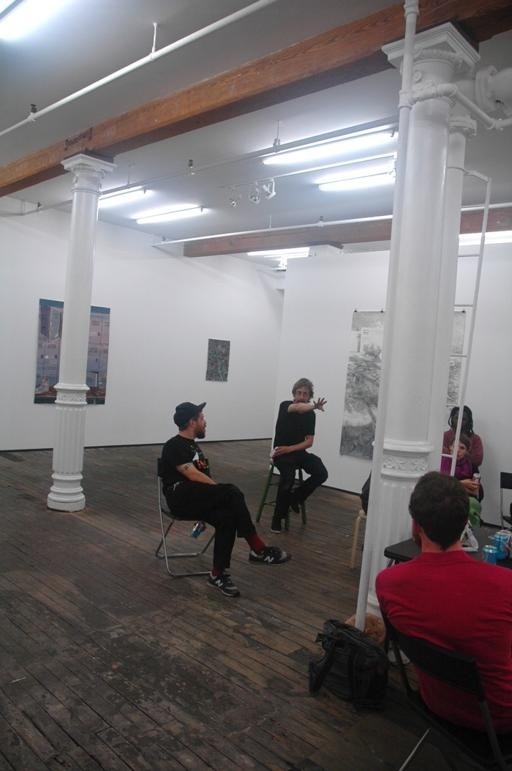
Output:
[249,547,292,566]
[205,572,239,597]
[291,495,300,514]
[271,517,282,534]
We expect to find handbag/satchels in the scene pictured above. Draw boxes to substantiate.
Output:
[307,621,387,713]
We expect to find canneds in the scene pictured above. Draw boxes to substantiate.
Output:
[192,521,205,538]
[481,544,498,565]
[473,473,481,483]
[495,530,511,561]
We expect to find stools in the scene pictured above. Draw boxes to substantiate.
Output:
[257,458,307,532]
[348,510,367,569]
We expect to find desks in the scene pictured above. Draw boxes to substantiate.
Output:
[384,522,512,571]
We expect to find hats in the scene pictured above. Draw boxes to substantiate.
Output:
[174,402,206,426]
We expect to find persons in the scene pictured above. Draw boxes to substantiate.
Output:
[442,435,482,530]
[442,406,484,502]
[271,377,328,534]
[375,472,512,739]
[161,402,290,597]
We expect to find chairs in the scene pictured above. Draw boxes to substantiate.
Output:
[498,472,512,532]
[380,607,512,771]
[152,456,216,577]
